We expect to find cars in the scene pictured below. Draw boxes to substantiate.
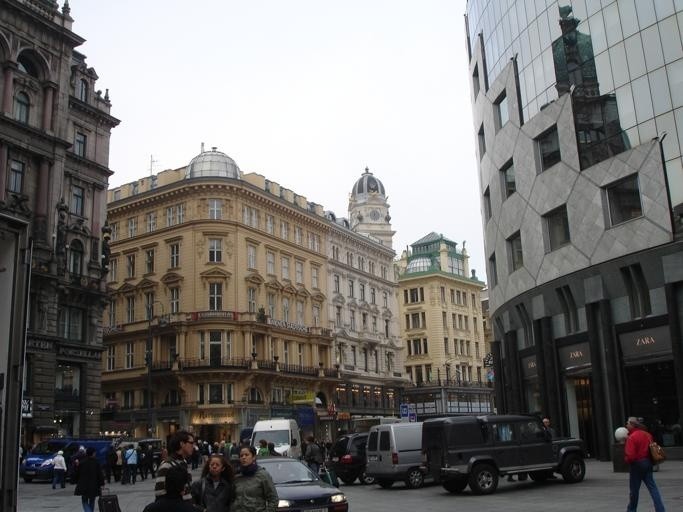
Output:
[253,458,348,512]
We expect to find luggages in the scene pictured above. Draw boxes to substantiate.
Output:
[98,488,121,511]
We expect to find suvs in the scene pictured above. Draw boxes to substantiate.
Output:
[117,438,164,473]
[418,413,588,497]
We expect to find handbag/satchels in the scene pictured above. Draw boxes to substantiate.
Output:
[649,442,667,464]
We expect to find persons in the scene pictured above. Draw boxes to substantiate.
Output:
[52,450,67,489]
[623,416,666,512]
[542,418,556,438]
[70,443,155,512]
[155,430,279,512]
[20,440,37,462]
[258,435,333,474]
[637,417,659,472]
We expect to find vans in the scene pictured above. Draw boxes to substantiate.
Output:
[22,437,113,482]
[239,415,434,489]
